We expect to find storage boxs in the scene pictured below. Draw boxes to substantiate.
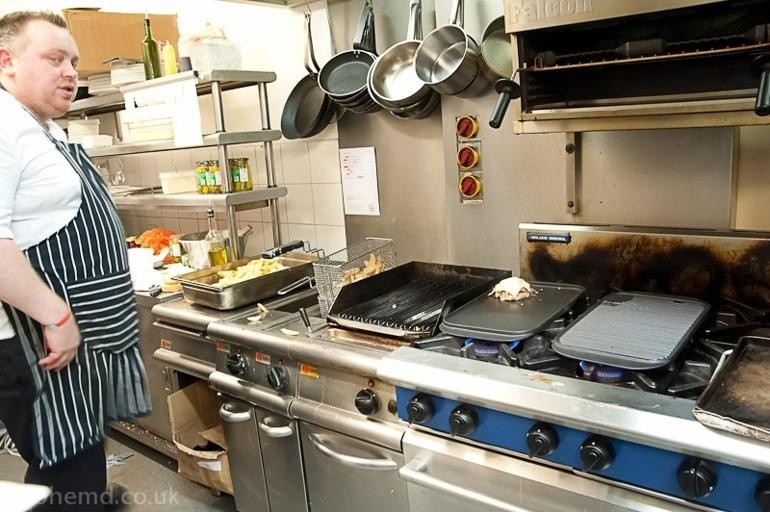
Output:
[119,106,173,145]
[59,7,181,77]
[166,377,234,496]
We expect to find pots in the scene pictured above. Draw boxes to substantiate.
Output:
[280,0,513,139]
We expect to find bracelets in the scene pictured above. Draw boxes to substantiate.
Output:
[43,312,71,330]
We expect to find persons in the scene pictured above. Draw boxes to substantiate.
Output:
[0,12,153,511]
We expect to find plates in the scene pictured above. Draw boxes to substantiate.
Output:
[67,118,113,151]
[88,63,145,96]
[0,479,51,512]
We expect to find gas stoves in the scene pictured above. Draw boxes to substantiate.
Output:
[376,287,770,512]
[207,262,511,452]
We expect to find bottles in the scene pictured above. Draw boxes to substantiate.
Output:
[163,40,177,75]
[142,13,161,80]
[205,200,228,266]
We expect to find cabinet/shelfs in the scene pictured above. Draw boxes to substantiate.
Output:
[52,69,287,211]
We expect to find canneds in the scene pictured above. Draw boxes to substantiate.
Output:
[195,158,253,194]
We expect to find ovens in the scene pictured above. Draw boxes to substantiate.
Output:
[489,1,769,136]
